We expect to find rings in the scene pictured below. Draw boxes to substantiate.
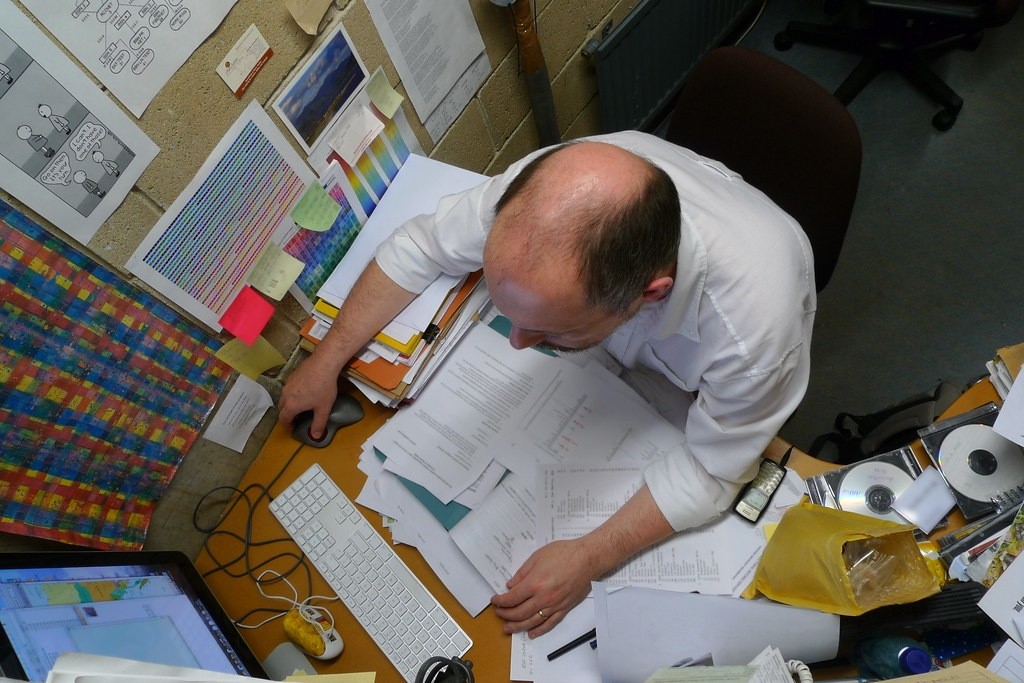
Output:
[539,611,547,619]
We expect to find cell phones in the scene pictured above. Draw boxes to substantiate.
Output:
[732,457,788,524]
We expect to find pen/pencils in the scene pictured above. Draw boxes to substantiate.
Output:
[547,628,597,662]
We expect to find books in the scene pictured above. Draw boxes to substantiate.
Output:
[300,152,495,410]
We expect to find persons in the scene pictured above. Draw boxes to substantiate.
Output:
[276,128,818,641]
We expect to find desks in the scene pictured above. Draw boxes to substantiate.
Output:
[191,375,1004,683]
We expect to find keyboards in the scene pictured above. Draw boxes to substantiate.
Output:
[268,463,474,683]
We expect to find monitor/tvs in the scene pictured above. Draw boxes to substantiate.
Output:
[0,550,270,683]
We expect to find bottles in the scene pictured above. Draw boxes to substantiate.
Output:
[860,635,932,679]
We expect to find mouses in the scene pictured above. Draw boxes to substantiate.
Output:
[289,394,365,448]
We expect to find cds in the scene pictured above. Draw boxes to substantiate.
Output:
[937,424,1024,502]
[839,461,915,526]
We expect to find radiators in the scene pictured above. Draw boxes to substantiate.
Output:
[579,0,755,131]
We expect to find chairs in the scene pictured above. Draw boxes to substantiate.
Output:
[774,0,986,129]
[667,45,862,289]
